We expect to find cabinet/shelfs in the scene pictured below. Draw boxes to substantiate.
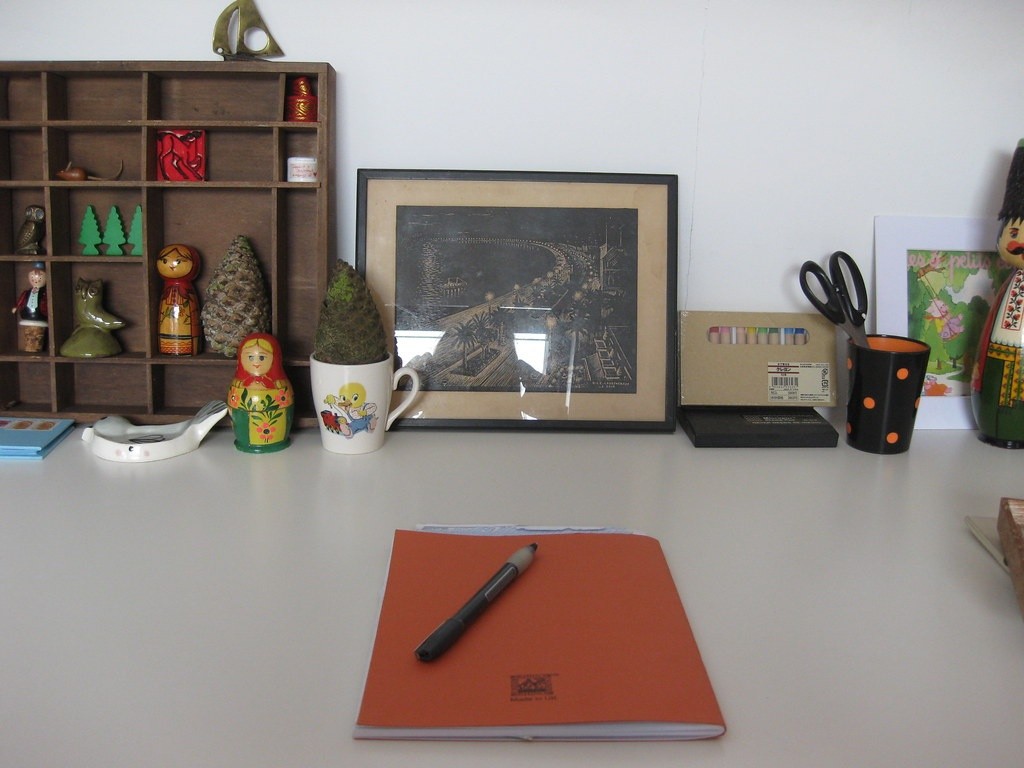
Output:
[0,59,336,429]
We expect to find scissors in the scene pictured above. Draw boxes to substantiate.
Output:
[799,251,869,348]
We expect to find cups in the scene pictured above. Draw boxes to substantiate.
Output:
[310,352,420,455]
[847,334,931,454]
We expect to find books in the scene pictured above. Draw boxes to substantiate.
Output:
[351,524,727,741]
[0,416,77,462]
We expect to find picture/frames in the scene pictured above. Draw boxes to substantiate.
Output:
[356,167,681,434]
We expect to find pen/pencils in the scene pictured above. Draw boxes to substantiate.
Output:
[411,541,539,662]
